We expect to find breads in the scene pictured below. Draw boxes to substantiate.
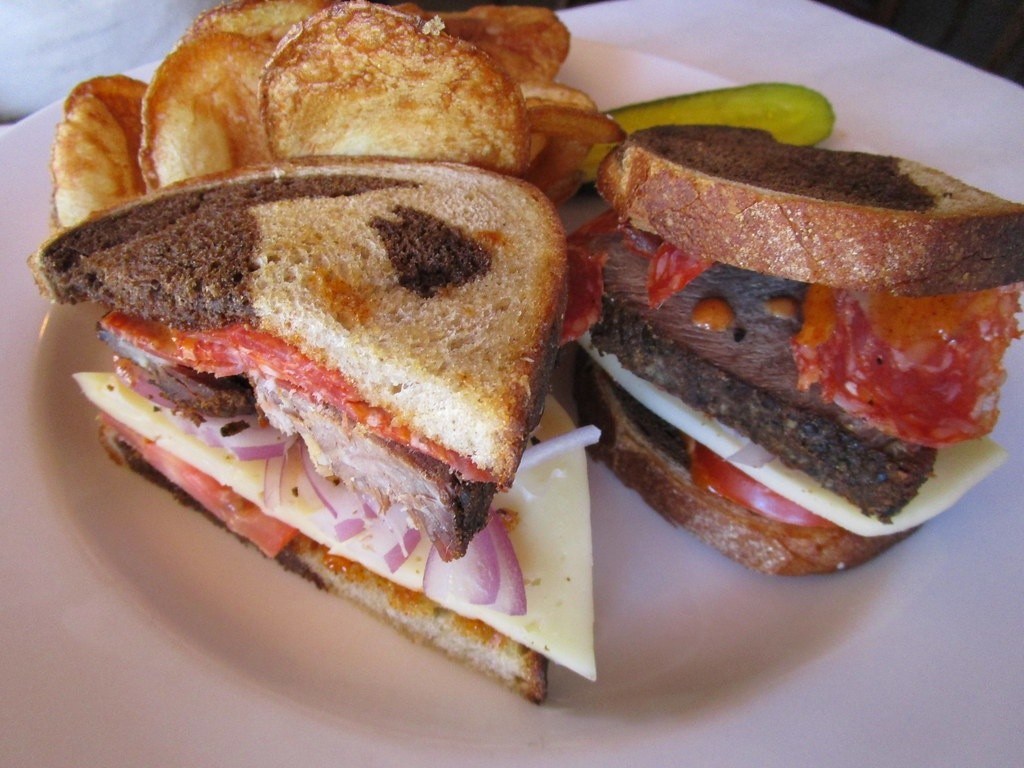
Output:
[52,0,594,235]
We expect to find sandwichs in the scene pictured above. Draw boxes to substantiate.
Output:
[29,161,597,705]
[566,132,1024,576]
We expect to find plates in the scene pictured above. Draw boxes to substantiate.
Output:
[0,0,1024,768]
